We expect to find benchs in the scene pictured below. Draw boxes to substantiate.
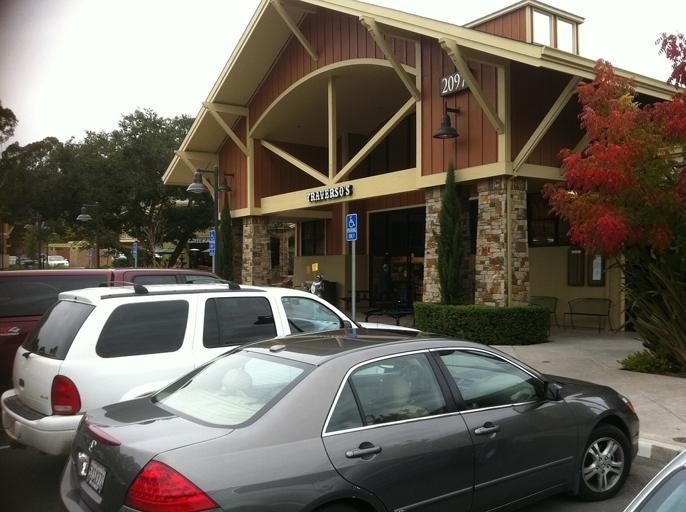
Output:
[529,294,613,335]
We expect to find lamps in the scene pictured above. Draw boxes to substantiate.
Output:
[433,101,460,139]
[186,169,234,194]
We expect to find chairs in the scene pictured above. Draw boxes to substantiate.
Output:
[380,373,428,420]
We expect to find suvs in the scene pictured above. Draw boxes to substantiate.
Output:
[0,263,233,396]
[0,280,423,476]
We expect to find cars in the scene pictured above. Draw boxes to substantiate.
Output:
[624,446,686,512]
[51,323,641,512]
[8,255,71,269]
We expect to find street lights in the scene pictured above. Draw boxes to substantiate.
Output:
[185,165,220,277]
[75,200,102,269]
